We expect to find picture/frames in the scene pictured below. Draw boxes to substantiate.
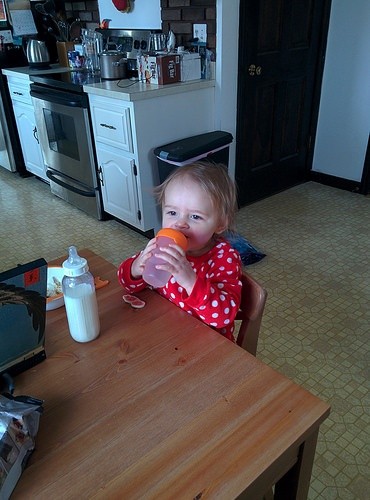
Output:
[0,0,7,22]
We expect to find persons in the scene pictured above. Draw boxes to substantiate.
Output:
[22,0,84,63]
[117,162,242,342]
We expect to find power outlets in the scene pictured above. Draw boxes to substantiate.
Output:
[192,23,207,43]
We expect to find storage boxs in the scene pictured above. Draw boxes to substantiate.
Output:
[0,257,50,385]
[180,53,202,83]
[136,53,180,85]
[55,41,79,67]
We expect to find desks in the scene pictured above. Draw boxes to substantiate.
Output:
[0,247,333,500]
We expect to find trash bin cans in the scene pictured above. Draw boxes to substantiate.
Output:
[154,129,234,186]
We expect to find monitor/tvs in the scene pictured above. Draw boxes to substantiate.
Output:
[0,257,48,394]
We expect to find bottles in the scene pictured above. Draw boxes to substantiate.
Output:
[142,228,188,287]
[61,246,99,343]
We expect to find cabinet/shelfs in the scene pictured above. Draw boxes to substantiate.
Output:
[88,86,216,240]
[6,75,50,186]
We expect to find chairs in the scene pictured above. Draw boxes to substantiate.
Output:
[234,271,269,358]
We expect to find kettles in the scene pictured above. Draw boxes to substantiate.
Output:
[21,35,50,68]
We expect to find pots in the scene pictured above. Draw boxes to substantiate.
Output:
[97,46,129,79]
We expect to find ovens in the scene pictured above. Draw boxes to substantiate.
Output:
[25,83,100,220]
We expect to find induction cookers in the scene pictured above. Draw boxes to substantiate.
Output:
[29,24,164,93]
